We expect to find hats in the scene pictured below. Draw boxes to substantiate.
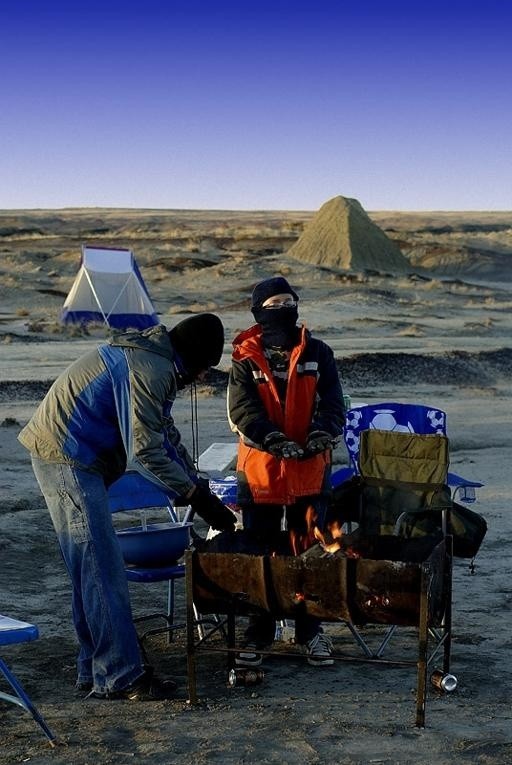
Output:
[252,278,300,345]
[170,315,224,385]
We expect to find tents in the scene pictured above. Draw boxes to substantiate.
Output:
[61,245,161,332]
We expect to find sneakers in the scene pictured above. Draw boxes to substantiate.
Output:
[306,632,336,667]
[234,641,275,664]
[78,666,175,700]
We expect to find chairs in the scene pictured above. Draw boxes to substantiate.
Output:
[335,399,483,505]
[103,471,231,665]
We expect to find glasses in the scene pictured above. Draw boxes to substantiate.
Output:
[263,301,297,310]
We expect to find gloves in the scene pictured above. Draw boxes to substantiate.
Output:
[265,430,302,459]
[306,431,336,456]
[189,480,236,535]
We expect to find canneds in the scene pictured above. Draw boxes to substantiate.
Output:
[430,669,458,693]
[227,667,267,688]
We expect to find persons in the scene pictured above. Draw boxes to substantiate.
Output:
[15,312,237,701]
[226,276,345,667]
